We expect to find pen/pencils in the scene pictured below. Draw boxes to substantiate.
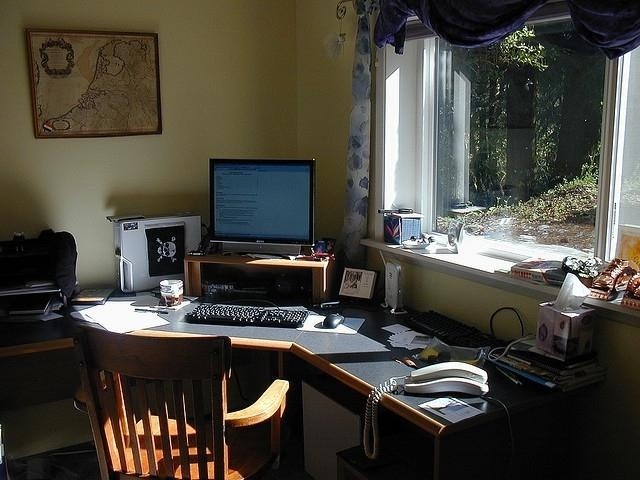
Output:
[150,306,177,311]
[504,369,523,384]
[295,256,321,261]
[135,309,168,314]
[495,366,519,384]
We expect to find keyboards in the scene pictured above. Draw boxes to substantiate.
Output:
[408,309,500,349]
[185,301,308,329]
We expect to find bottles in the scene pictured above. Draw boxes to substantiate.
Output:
[160,279,184,306]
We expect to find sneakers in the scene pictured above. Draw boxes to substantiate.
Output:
[591,258,640,309]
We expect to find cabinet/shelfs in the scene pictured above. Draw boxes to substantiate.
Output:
[1,331,96,463]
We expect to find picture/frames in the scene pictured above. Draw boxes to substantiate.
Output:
[23,27,163,139]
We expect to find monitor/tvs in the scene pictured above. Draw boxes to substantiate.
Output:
[209,158,316,256]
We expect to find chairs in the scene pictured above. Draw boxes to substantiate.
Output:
[76,324,290,479]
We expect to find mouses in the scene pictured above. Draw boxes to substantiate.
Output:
[323,312,344,329]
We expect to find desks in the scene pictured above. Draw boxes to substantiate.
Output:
[70,253,606,479]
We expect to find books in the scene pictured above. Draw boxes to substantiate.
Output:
[70,286,116,306]
[497,332,613,394]
[512,255,582,286]
[1,274,65,324]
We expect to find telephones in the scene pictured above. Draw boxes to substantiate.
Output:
[403,361,490,397]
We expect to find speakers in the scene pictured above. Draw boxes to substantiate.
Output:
[384,259,408,313]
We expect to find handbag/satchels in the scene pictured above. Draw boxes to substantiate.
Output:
[0,229,77,310]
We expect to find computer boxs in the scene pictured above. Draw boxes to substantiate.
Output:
[335,425,424,478]
[114,215,203,293]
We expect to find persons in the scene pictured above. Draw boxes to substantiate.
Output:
[343,272,361,290]
